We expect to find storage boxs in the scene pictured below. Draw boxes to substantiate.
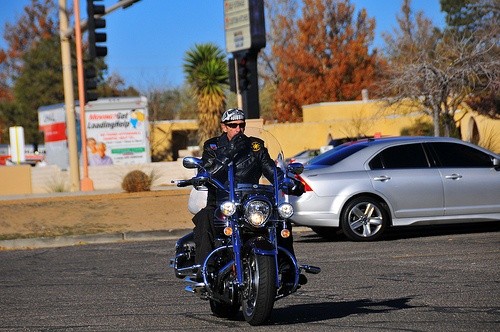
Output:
[188,186,208,214]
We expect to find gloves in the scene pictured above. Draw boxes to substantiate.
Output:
[282,178,304,196]
[222,131,251,159]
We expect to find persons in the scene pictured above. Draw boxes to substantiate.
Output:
[87,137,114,165]
[190,108,307,287]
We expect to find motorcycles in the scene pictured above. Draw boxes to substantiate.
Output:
[169,126,321,328]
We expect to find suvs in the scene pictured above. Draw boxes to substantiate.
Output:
[322,133,374,155]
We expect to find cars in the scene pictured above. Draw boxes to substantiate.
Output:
[277,137,500,244]
[285,148,320,168]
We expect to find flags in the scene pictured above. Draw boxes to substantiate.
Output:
[274,151,284,166]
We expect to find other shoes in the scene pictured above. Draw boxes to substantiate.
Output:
[195,266,211,283]
[281,271,307,285]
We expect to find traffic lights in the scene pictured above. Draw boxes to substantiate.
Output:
[84,66,99,105]
[236,56,249,92]
[86,0,108,61]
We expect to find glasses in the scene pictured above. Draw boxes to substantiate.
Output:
[225,123,245,128]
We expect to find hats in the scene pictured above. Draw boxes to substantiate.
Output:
[221,108,245,122]
